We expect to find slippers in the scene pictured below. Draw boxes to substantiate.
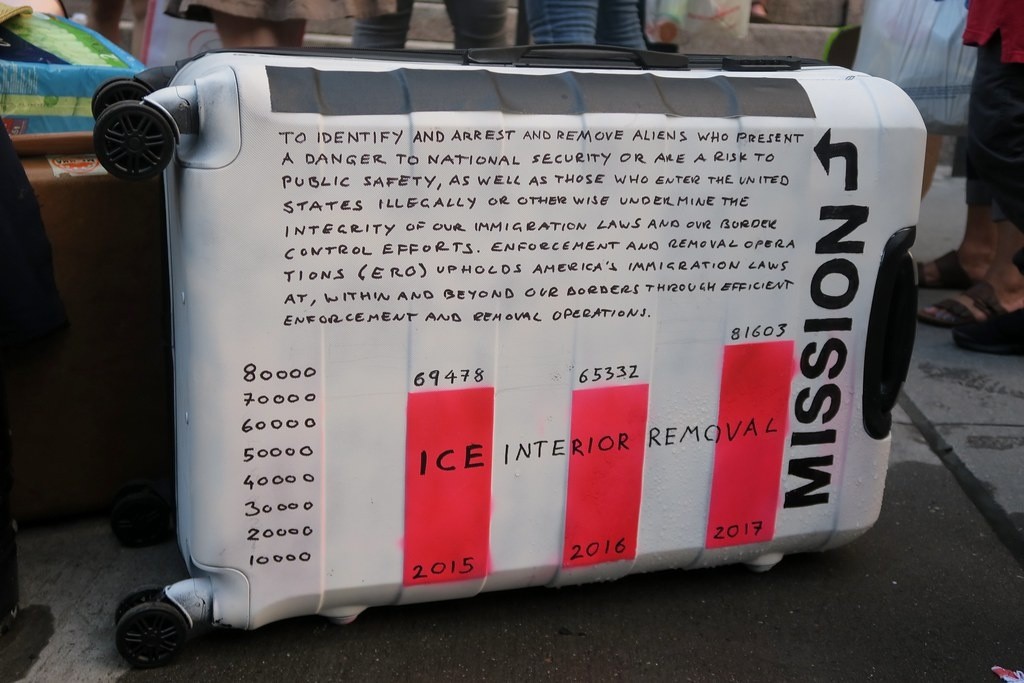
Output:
[918,250,974,289]
[916,280,1004,327]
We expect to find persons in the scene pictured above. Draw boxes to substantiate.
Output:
[162,0,399,52]
[516,0,653,51]
[850,0,1024,356]
[0,123,72,631]
[351,0,520,56]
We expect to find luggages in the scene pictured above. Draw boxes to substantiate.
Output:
[89,40,928,668]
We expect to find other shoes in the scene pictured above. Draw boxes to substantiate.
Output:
[950,304,1024,354]
